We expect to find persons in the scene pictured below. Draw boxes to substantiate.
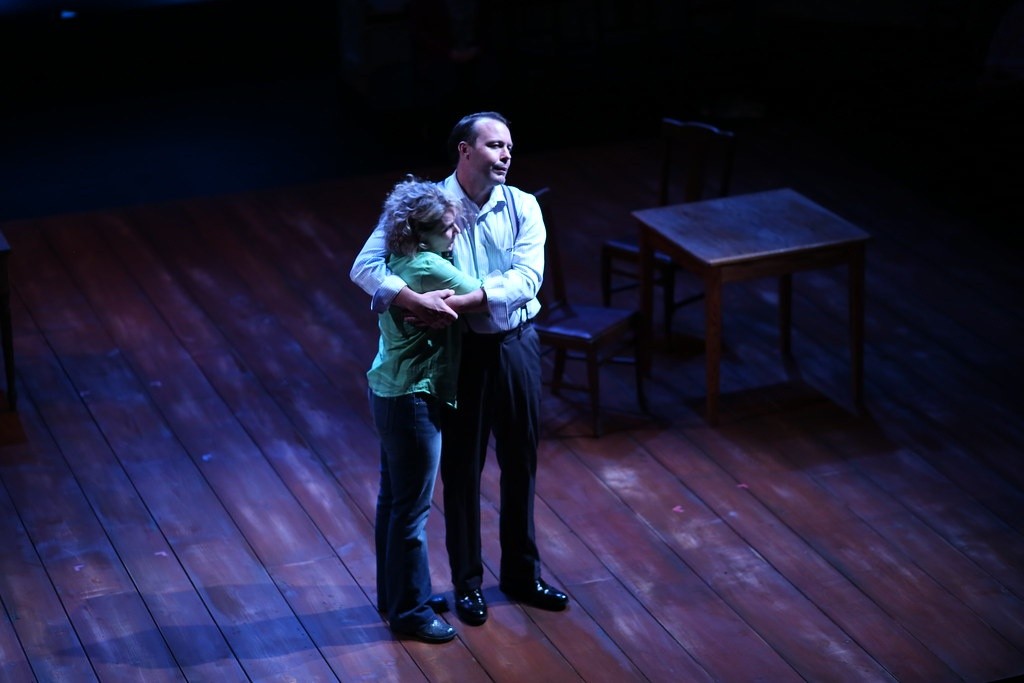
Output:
[350,111,569,645]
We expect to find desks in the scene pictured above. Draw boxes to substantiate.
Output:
[633,187,872,423]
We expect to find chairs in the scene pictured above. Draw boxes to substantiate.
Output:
[498,187,649,437]
[600,117,735,346]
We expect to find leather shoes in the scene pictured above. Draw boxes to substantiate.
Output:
[499,578,569,612]
[454,581,488,627]
[377,595,460,645]
